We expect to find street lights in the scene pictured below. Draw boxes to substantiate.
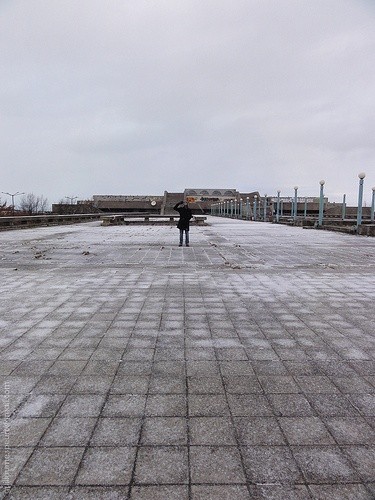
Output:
[244,202,246,218]
[317,179,326,227]
[249,202,252,218]
[246,196,250,221]
[66,196,79,205]
[292,184,299,224]
[276,188,281,222]
[281,199,283,217]
[271,199,275,217]
[210,199,239,217]
[240,197,243,219]
[341,194,346,220]
[304,196,308,218]
[263,193,267,219]
[370,187,375,222]
[258,201,261,218]
[253,195,258,221]
[354,172,366,228]
[1,191,23,212]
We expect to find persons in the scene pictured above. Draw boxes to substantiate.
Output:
[173,200,192,247]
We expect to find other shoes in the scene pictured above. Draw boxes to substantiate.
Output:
[179,243,183,247]
[186,243,190,247]
